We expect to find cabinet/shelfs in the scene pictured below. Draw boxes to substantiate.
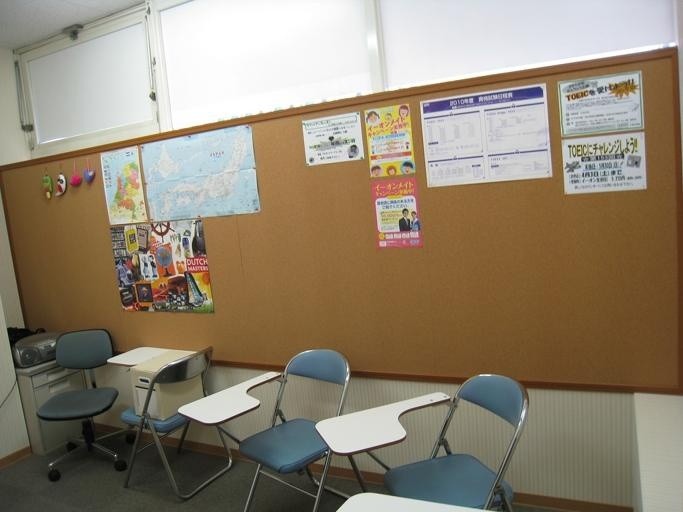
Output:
[13,363,91,453]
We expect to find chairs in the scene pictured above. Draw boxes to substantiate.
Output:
[37,329,530,512]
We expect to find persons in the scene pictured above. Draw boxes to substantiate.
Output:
[410,211,420,231]
[348,145,357,157]
[141,255,150,278]
[399,208,410,230]
[141,287,152,302]
[148,255,157,277]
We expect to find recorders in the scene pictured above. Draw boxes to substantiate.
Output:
[14,330,71,368]
